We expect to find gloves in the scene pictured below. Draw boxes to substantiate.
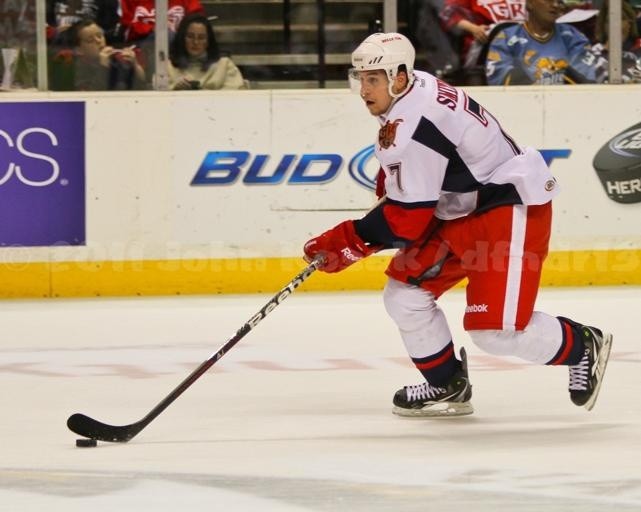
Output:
[302,219,382,274]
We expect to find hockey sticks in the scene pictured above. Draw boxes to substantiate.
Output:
[66,255,325,442]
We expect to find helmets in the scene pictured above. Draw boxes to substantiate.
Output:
[348,31,416,100]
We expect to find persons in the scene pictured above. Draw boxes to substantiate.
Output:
[405,1,641,87]
[0,1,251,92]
[300,32,607,409]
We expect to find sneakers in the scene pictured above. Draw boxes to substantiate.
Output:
[555,316,604,406]
[393,346,472,411]
